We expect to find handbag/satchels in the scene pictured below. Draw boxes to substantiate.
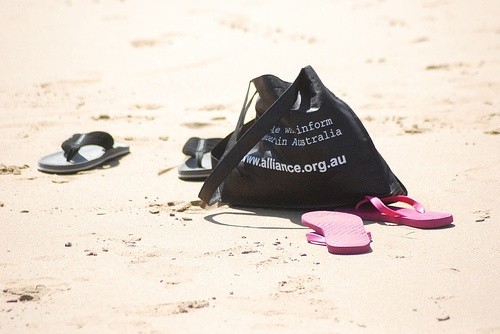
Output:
[194,63,411,210]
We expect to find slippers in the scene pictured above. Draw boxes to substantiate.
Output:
[176,135,225,181]
[39,129,131,175]
[299,207,373,255]
[346,193,454,231]
